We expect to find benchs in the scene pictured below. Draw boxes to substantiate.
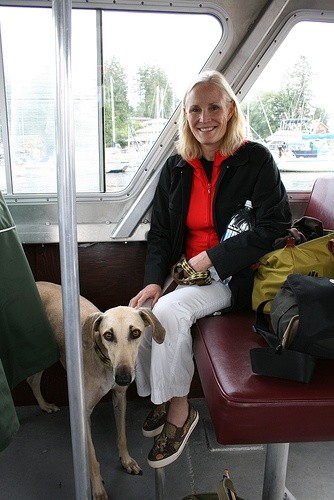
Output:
[193,178,334,500]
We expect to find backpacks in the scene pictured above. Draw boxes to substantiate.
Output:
[250,271,334,384]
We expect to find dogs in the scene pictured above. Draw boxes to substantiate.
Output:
[27,280,166,500]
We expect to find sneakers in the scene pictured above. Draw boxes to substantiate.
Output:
[148,399,197,468]
[142,398,173,437]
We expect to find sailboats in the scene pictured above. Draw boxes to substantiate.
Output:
[104,76,129,172]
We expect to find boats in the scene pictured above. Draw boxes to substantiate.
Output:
[291,149,318,159]
[267,118,310,148]
[129,118,170,164]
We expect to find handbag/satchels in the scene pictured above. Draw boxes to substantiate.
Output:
[251,215,334,315]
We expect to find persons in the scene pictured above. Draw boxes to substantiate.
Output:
[128,69,294,469]
